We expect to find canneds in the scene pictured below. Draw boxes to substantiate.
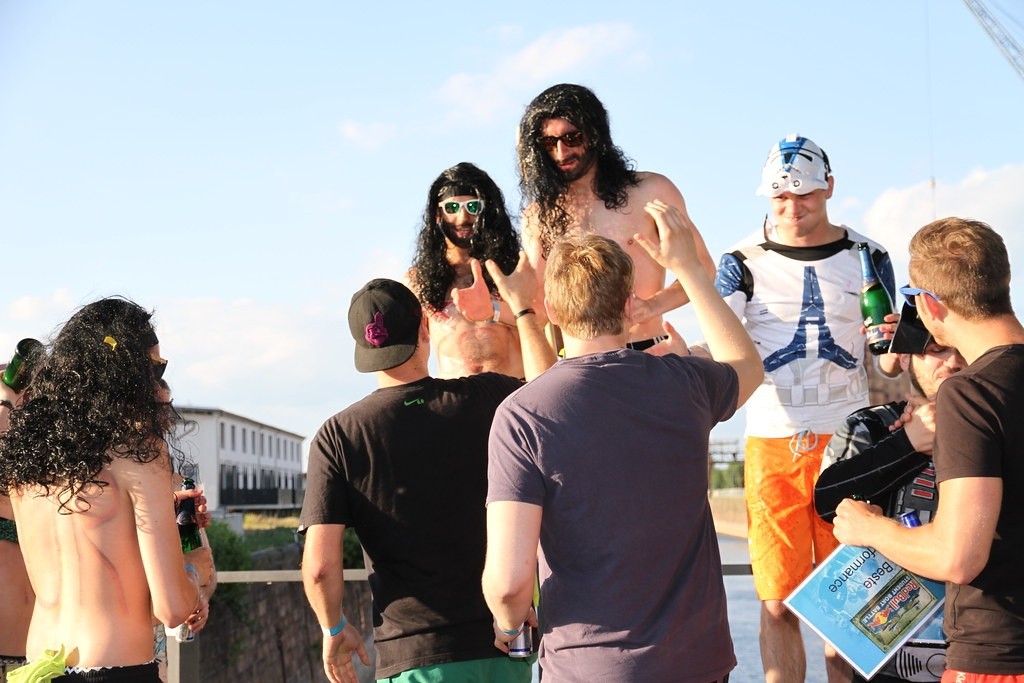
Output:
[176,621,196,643]
[896,510,922,527]
[507,622,533,658]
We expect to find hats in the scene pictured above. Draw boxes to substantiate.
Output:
[348,278,422,373]
[756,133,833,197]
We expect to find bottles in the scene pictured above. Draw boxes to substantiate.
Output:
[175,477,202,554]
[857,242,895,356]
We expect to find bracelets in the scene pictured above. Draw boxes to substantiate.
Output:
[514,308,535,320]
[322,614,346,636]
[688,349,694,358]
[186,565,199,579]
[501,622,524,635]
[490,299,500,322]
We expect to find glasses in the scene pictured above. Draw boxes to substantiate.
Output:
[537,131,585,152]
[438,200,486,215]
[899,284,941,306]
[150,353,168,381]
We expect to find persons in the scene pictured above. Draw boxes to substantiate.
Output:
[481,200,771,683]
[296,248,563,683]
[644,136,901,683]
[0,298,217,683]
[814,217,1024,683]
[400,162,522,379]
[517,83,717,355]
